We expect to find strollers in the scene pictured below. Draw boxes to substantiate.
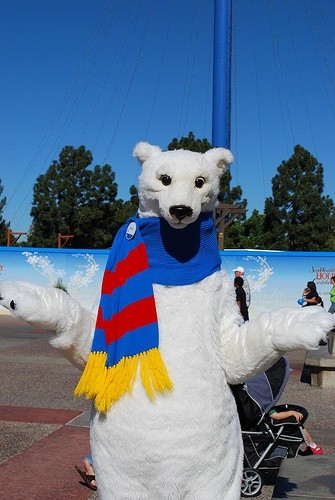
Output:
[229,356,308,497]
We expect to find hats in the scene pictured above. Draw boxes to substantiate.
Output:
[233,266,244,273]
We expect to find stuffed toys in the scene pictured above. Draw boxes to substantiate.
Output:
[0,141,335,500]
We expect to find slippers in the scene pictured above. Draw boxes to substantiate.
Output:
[73,464,99,489]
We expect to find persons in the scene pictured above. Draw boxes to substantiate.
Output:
[297,281,324,308]
[269,408,325,456]
[232,266,251,322]
[328,276,335,314]
[75,454,98,490]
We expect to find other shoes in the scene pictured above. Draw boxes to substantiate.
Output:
[300,444,323,455]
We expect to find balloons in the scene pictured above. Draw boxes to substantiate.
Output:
[298,299,303,305]
